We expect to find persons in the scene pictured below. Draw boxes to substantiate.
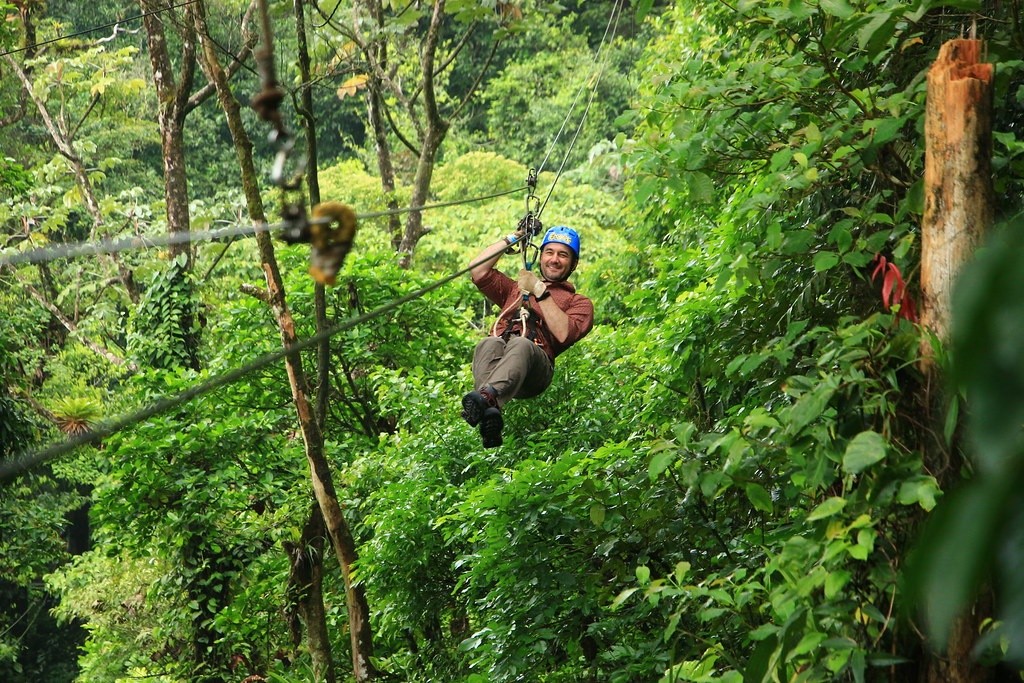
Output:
[462,225,594,448]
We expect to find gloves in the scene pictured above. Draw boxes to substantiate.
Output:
[518,271,547,298]
[520,219,542,236]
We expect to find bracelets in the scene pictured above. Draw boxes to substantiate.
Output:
[534,280,551,303]
[503,235,517,245]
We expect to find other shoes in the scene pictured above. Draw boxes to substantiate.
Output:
[480,408,502,448]
[462,388,496,426]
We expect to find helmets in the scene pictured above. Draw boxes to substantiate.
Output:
[541,226,580,256]
